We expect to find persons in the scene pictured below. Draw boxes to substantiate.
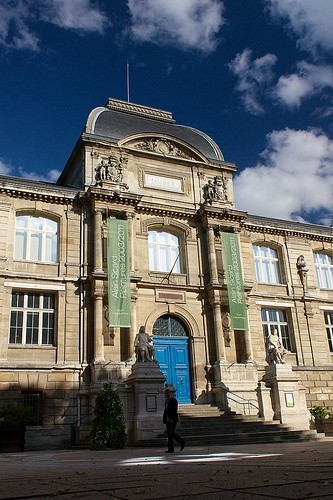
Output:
[268,328,288,364]
[142,137,181,156]
[97,156,124,183]
[205,175,229,200]
[162,388,185,453]
[133,326,157,363]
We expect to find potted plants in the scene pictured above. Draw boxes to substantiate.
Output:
[88,380,129,452]
[0,400,37,448]
[309,405,333,438]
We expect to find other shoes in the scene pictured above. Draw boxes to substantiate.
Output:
[180,440,185,451]
[165,450,174,453]
